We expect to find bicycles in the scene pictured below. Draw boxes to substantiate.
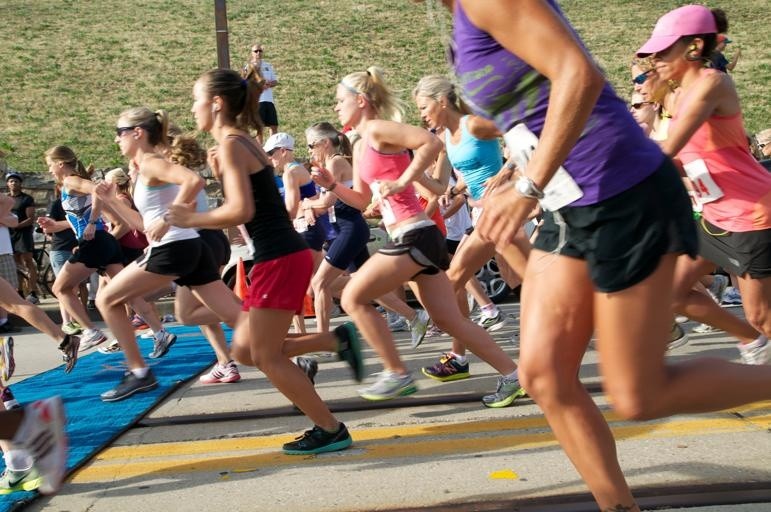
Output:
[19,227,59,298]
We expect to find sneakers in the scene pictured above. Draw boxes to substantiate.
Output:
[1,386,64,495]
[100,368,159,402]
[337,294,505,348]
[483,376,526,408]
[666,274,770,364]
[420,352,470,382]
[291,356,318,412]
[199,359,240,384]
[26,294,40,305]
[0,335,15,381]
[333,321,362,383]
[360,369,419,400]
[57,299,176,374]
[283,423,351,454]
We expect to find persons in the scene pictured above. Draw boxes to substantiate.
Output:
[410,2,771,510]
[1,437,43,493]
[0,108,318,402]
[308,67,529,408]
[638,2,771,364]
[1,394,69,495]
[244,46,535,380]
[631,58,771,352]
[162,67,367,455]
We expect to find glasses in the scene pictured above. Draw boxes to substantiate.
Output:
[632,69,653,84]
[254,50,263,53]
[757,141,770,147]
[632,101,653,109]
[116,126,135,136]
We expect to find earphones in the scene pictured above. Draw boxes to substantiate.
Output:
[690,44,698,52]
[133,132,138,138]
[212,103,219,112]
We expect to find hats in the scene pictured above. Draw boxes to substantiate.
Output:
[263,132,294,152]
[5,172,24,181]
[717,35,733,43]
[636,4,719,58]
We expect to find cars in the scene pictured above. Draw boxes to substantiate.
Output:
[221,218,522,311]
